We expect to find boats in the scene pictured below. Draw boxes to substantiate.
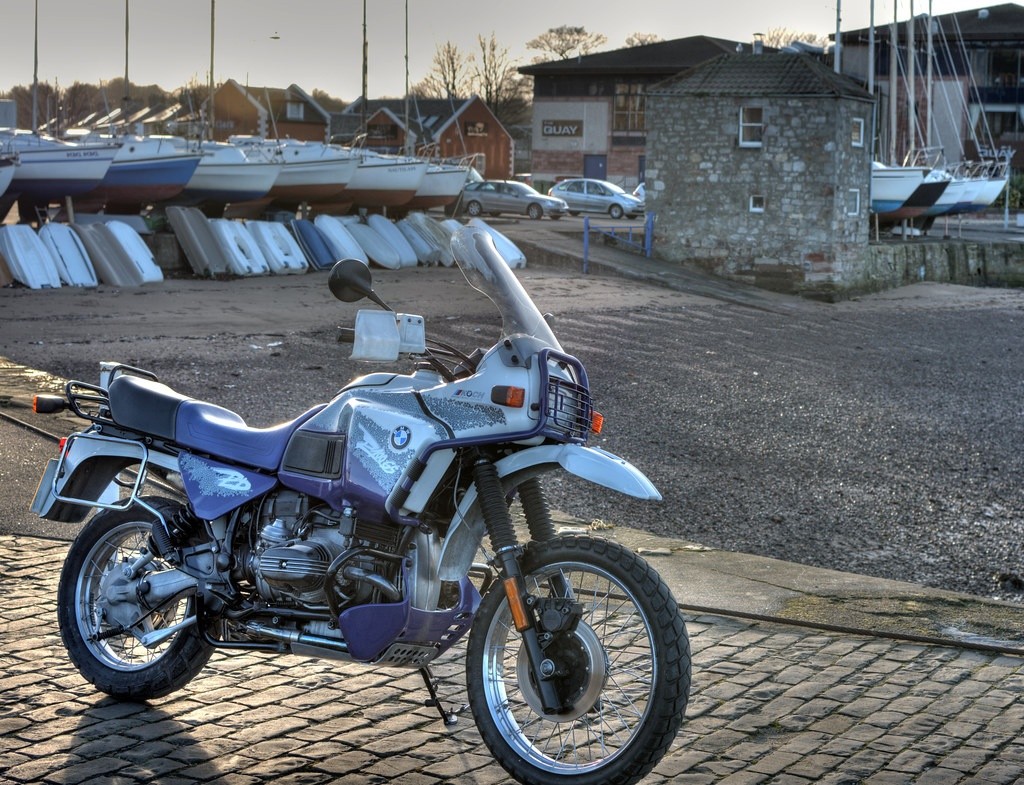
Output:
[396,212,526,269]
[291,219,339,271]
[244,220,309,275]
[366,214,419,267]
[73,220,143,285]
[348,222,402,269]
[165,206,237,280]
[105,221,165,283]
[209,216,271,277]
[313,215,369,266]
[1,223,61,289]
[39,223,98,286]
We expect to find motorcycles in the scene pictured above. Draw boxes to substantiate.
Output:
[28,225,691,785]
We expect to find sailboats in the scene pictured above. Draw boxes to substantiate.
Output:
[90,0,286,204]
[0,1,125,223]
[228,0,478,210]
[833,0,1010,226]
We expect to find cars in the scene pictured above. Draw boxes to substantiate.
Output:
[548,179,644,219]
[463,180,568,221]
[633,182,645,203]
